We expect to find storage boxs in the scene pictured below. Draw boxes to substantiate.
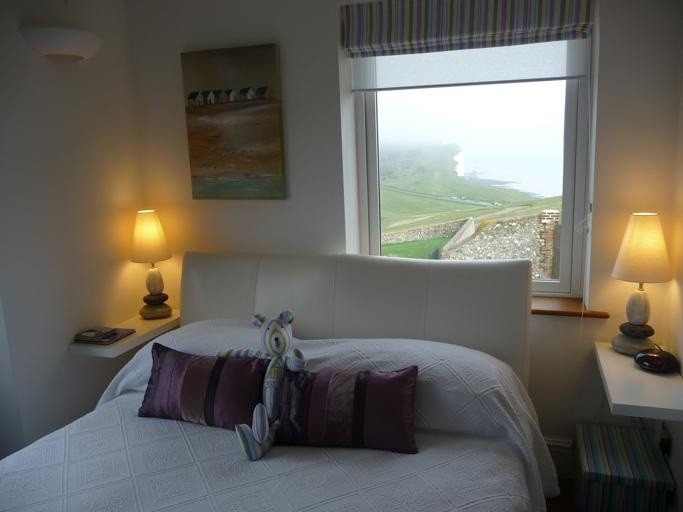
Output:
[573,423,676,512]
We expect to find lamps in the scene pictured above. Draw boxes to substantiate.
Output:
[611,213,672,356]
[130,210,172,320]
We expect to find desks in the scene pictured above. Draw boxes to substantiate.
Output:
[71,309,181,359]
[593,341,683,422]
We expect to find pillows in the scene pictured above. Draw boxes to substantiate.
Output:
[277,364,420,454]
[1,251,560,512]
[137,342,270,432]
[115,320,516,438]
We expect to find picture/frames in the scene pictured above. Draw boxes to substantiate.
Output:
[180,43,289,199]
